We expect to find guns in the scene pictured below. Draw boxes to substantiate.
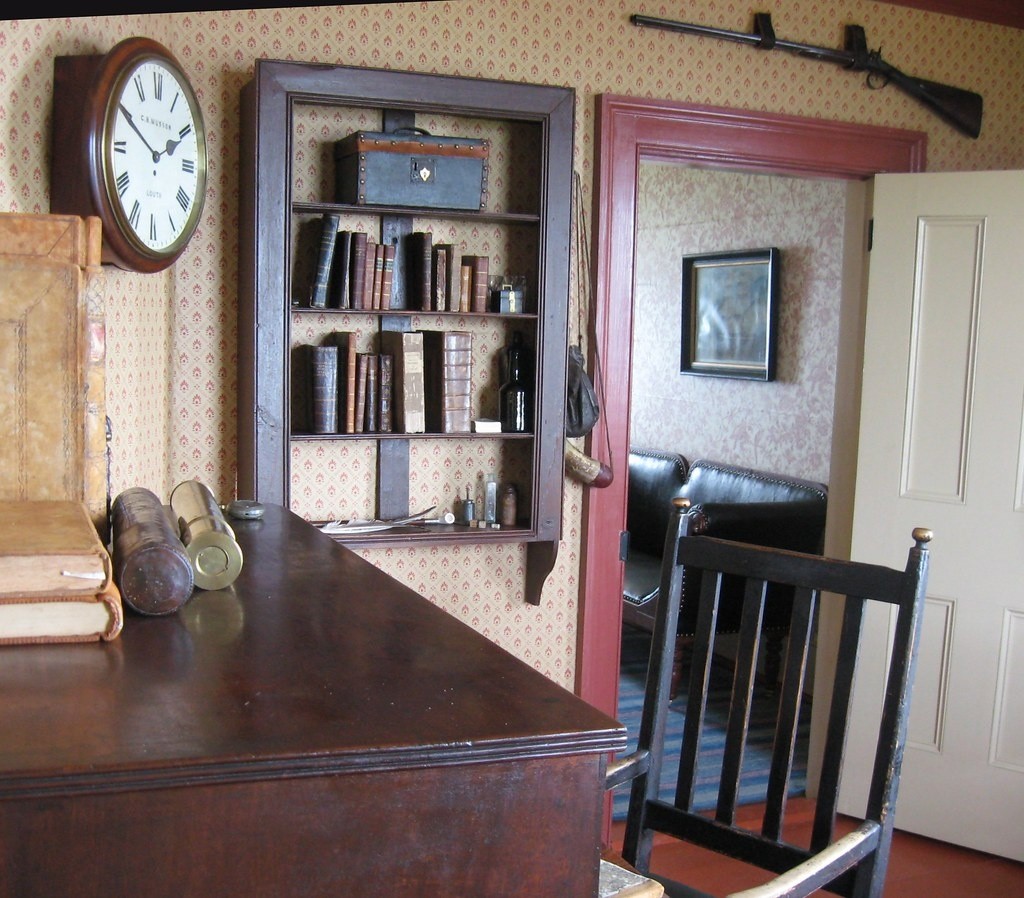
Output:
[630,13,985,140]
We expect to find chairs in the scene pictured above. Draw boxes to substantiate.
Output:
[604,496,933,898]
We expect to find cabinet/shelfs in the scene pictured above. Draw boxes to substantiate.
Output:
[241,60,577,603]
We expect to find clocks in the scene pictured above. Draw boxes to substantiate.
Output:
[83,34,208,272]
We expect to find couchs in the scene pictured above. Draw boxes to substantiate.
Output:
[621,446,828,707]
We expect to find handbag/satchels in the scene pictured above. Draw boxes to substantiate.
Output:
[565,343,600,438]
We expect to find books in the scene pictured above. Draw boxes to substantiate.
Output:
[311,215,501,433]
[0,499,124,646]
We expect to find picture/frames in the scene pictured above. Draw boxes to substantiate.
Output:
[679,247,780,382]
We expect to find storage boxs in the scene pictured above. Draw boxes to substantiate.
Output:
[333,130,493,210]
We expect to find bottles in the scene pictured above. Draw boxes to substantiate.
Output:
[500,352,533,433]
[500,331,533,387]
[502,483,516,526]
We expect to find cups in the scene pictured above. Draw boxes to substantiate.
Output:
[486,275,503,311]
[505,276,527,312]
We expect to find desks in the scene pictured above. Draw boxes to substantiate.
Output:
[1,489,630,897]
